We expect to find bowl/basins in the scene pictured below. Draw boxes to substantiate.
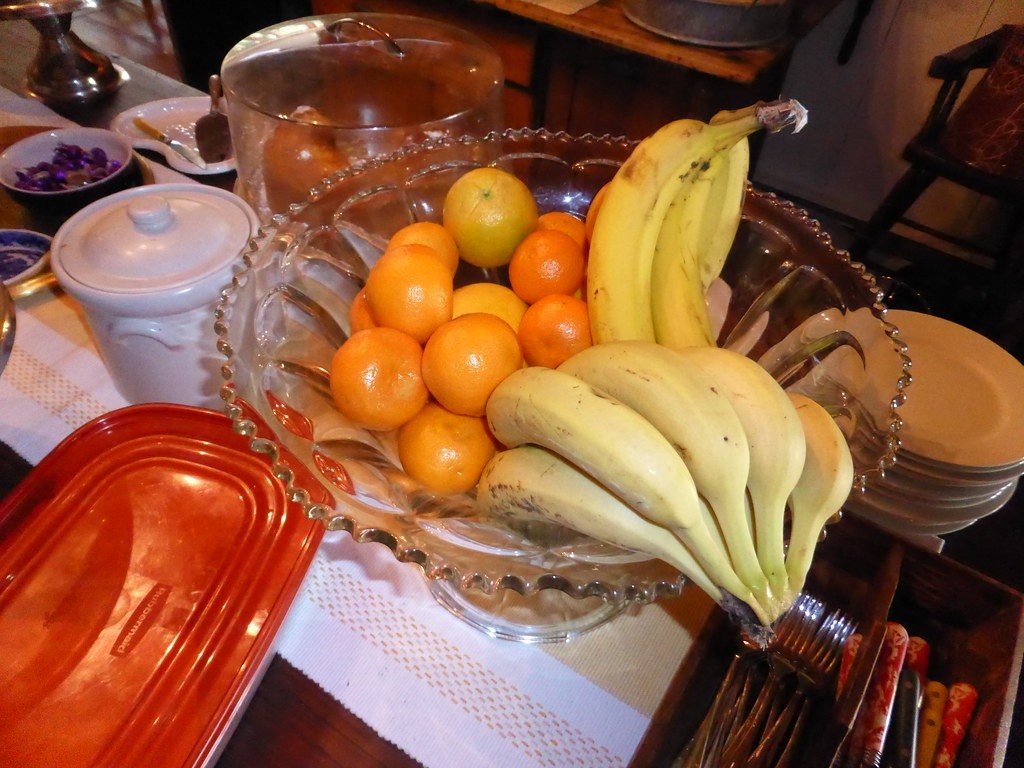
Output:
[215,124,914,651]
[222,14,506,248]
[0,125,136,220]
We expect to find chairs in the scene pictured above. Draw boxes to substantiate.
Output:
[842,23,1023,367]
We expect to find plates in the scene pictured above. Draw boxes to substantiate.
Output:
[110,96,237,178]
[757,305,1023,551]
[0,226,56,289]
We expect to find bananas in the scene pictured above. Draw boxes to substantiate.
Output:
[477,102,855,631]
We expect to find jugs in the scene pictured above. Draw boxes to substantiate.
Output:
[0,2,123,115]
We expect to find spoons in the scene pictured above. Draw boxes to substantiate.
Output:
[196,75,231,164]
[668,587,862,768]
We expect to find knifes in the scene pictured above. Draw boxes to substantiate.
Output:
[132,115,207,171]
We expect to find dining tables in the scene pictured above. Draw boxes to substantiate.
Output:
[0,11,1024,768]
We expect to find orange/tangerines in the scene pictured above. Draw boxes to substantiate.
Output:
[326,167,592,501]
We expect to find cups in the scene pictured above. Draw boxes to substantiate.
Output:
[48,182,268,416]
